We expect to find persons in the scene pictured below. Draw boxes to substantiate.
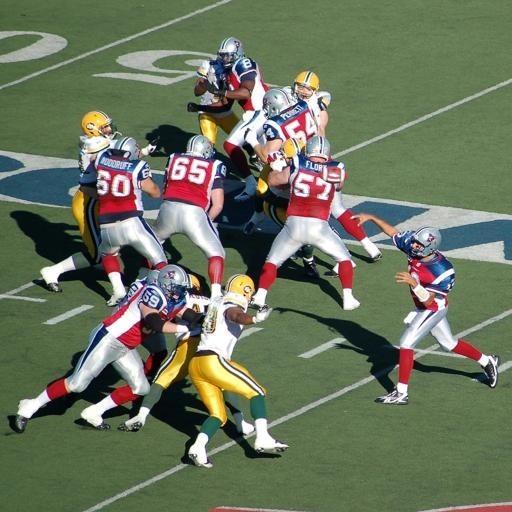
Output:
[187,36,382,312]
[13,264,205,434]
[153,133,225,299]
[38,112,168,306]
[119,271,256,435]
[186,275,290,467]
[349,213,501,406]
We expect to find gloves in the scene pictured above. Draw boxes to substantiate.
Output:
[252,304,273,324]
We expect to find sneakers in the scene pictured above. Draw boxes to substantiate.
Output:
[15,399,290,468]
[481,354,501,388]
[106,292,127,307]
[40,266,63,293]
[233,189,384,311]
[376,388,408,405]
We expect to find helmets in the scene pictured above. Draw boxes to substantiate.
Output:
[217,37,320,120]
[81,111,331,163]
[146,264,256,313]
[409,225,442,258]
